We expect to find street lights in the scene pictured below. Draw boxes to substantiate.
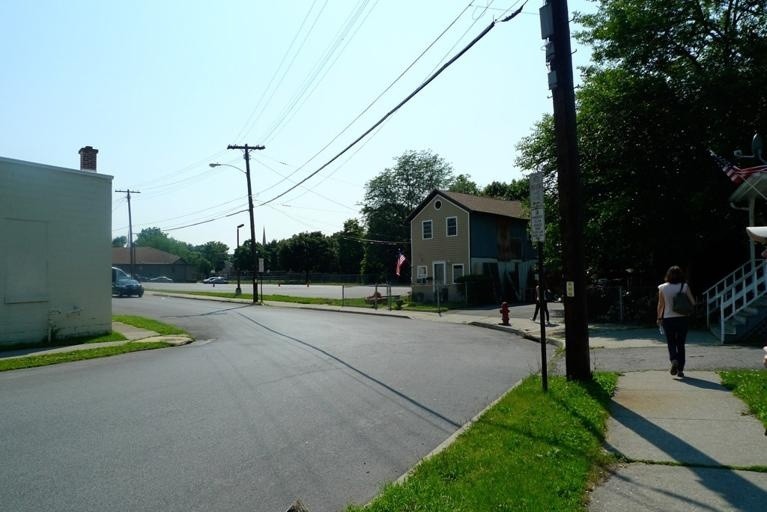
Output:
[209,161,261,305]
[235,222,246,294]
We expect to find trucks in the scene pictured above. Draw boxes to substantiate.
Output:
[112,266,133,284]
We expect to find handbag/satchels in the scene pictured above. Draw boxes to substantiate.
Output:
[672,292,693,315]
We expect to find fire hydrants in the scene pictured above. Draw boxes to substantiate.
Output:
[499,301,512,327]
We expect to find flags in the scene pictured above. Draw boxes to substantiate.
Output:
[395,249,406,276]
[712,152,766,186]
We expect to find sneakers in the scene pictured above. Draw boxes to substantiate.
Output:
[671,360,684,377]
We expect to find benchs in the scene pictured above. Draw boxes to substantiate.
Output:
[374,294,403,308]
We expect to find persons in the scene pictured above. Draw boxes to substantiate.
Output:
[656,264,694,379]
[530,281,551,326]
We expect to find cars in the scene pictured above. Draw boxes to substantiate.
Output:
[112,279,145,297]
[129,274,149,282]
[203,276,229,284]
[150,275,174,283]
